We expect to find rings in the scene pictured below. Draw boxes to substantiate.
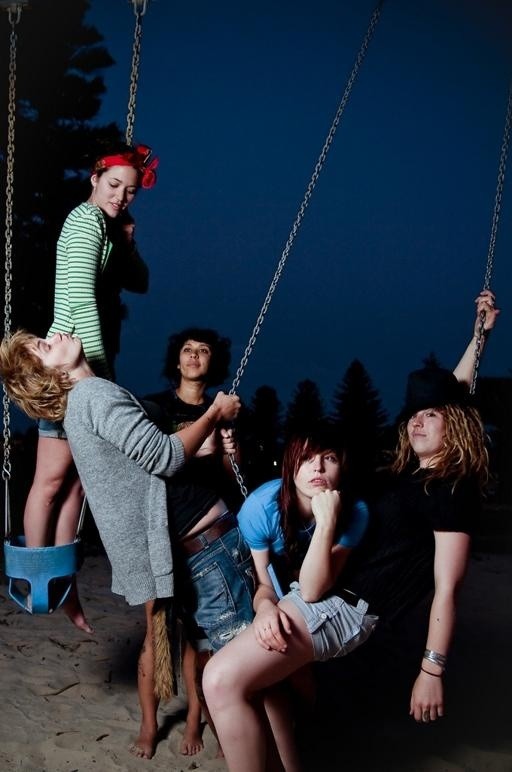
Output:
[421,709,429,723]
[264,627,271,631]
[486,296,495,306]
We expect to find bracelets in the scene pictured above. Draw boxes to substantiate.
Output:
[423,649,446,668]
[420,667,442,679]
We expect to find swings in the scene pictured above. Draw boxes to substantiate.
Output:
[0,2,148,614]
[222,0,510,673]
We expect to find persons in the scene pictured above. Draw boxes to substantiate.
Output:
[129,326,243,761]
[235,422,370,709]
[0,326,253,654]
[21,141,160,633]
[201,288,504,772]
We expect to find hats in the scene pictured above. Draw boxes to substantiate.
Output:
[393,370,464,422]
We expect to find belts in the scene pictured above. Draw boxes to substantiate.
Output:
[179,509,239,557]
[336,587,380,617]
